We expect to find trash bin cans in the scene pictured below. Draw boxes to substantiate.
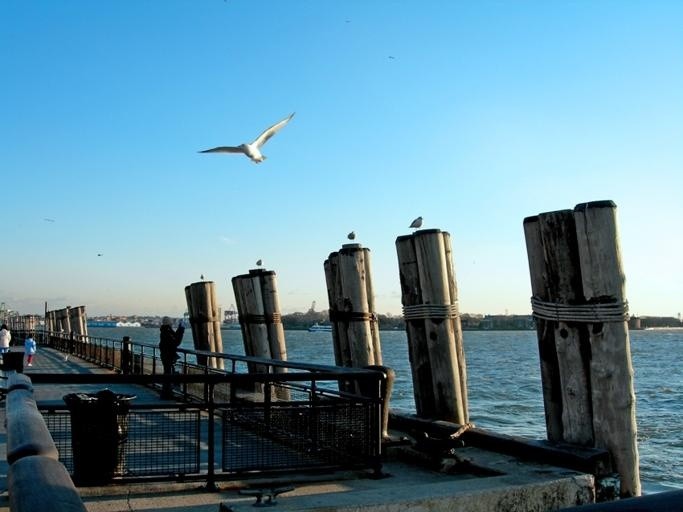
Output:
[1,352,25,373]
[63,392,137,488]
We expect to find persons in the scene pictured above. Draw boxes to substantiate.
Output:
[159,315,187,401]
[0,323,13,360]
[24,333,37,368]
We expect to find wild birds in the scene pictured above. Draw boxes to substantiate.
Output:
[409,215,424,230]
[200,274,203,281]
[348,231,355,243]
[198,110,297,163]
[256,259,262,268]
